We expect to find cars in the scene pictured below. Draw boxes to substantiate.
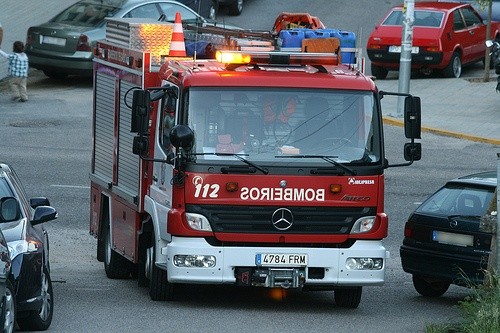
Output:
[366,1,500,80]
[24,0,249,80]
[399,171,498,298]
[0,159,59,333]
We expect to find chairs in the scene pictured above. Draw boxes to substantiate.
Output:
[192,92,227,146]
[458,194,483,213]
[226,109,263,146]
[295,97,343,149]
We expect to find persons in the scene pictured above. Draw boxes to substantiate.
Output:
[0,41,29,102]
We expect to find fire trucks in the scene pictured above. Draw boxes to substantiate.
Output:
[88,17,422,310]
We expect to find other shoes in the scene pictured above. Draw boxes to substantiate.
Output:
[12,96,19,101]
[18,99,27,102]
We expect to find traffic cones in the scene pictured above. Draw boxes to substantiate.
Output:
[167,11,187,58]
